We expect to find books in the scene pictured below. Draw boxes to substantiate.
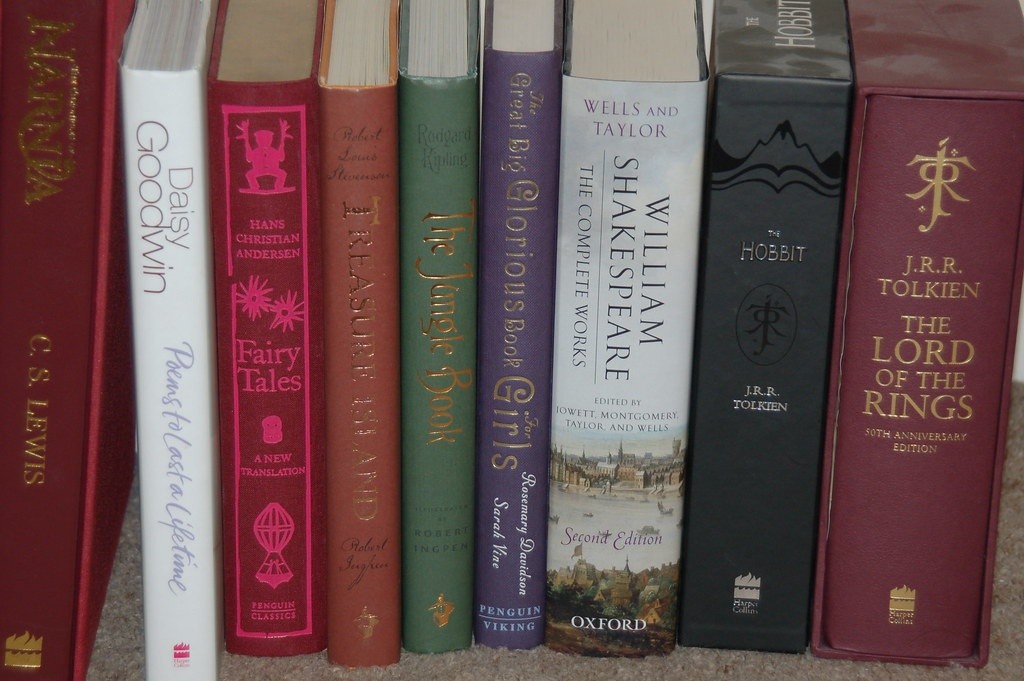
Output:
[810,0,1024,668]
[318,0,402,667]
[207,0,329,656]
[400,0,479,655]
[477,0,566,652]
[543,1,708,659]
[119,0,226,681]
[677,0,852,653]
[0,1,136,681]
